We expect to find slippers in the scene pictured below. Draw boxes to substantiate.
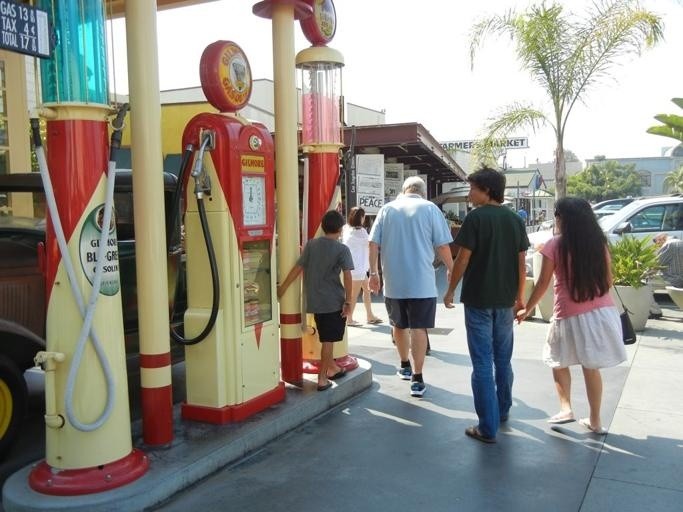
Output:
[547,412,576,423]
[368,317,383,324]
[347,321,364,326]
[328,366,346,380]
[579,418,606,434]
[500,416,509,423]
[465,425,497,443]
[317,381,332,391]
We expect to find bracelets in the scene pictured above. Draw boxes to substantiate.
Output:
[369,271,379,277]
[343,302,354,306]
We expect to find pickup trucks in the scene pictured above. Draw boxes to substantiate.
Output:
[0,167,187,463]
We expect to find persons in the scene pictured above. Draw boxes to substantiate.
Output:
[640,230,682,321]
[516,205,528,226]
[442,164,531,444]
[277,209,356,392]
[342,205,384,327]
[514,194,626,435]
[366,174,455,398]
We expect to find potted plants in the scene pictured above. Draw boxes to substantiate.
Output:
[607,231,669,332]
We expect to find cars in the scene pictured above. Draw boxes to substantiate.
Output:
[521,193,683,294]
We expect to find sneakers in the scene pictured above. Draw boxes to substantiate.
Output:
[410,381,426,396]
[396,367,413,380]
[647,310,662,319]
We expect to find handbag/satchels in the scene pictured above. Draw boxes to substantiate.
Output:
[619,311,636,345]
